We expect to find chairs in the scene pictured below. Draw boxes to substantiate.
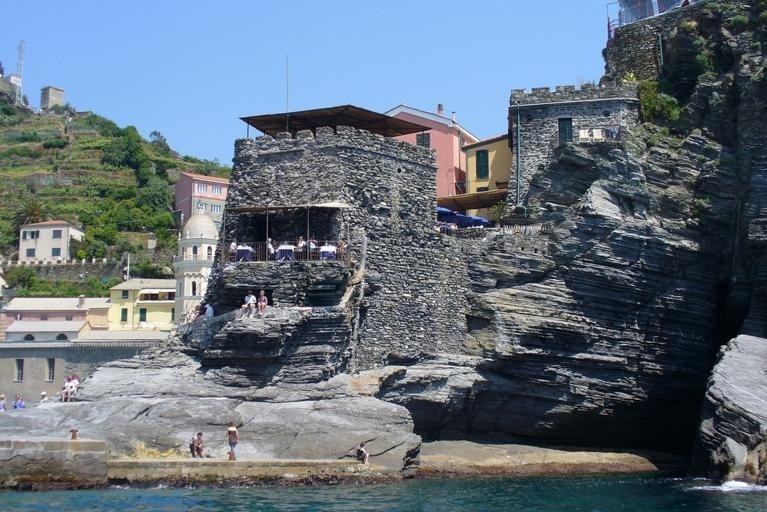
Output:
[64,375,80,397]
[227,243,343,261]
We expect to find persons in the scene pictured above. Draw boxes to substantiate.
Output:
[227,421,239,459]
[241,290,257,318]
[256,290,267,316]
[265,238,276,261]
[12,394,21,409]
[296,236,307,253]
[0,393,6,411]
[337,237,348,255]
[40,391,48,402]
[60,374,79,403]
[15,395,25,408]
[199,304,214,318]
[356,442,369,463]
[189,431,204,458]
[228,238,237,262]
